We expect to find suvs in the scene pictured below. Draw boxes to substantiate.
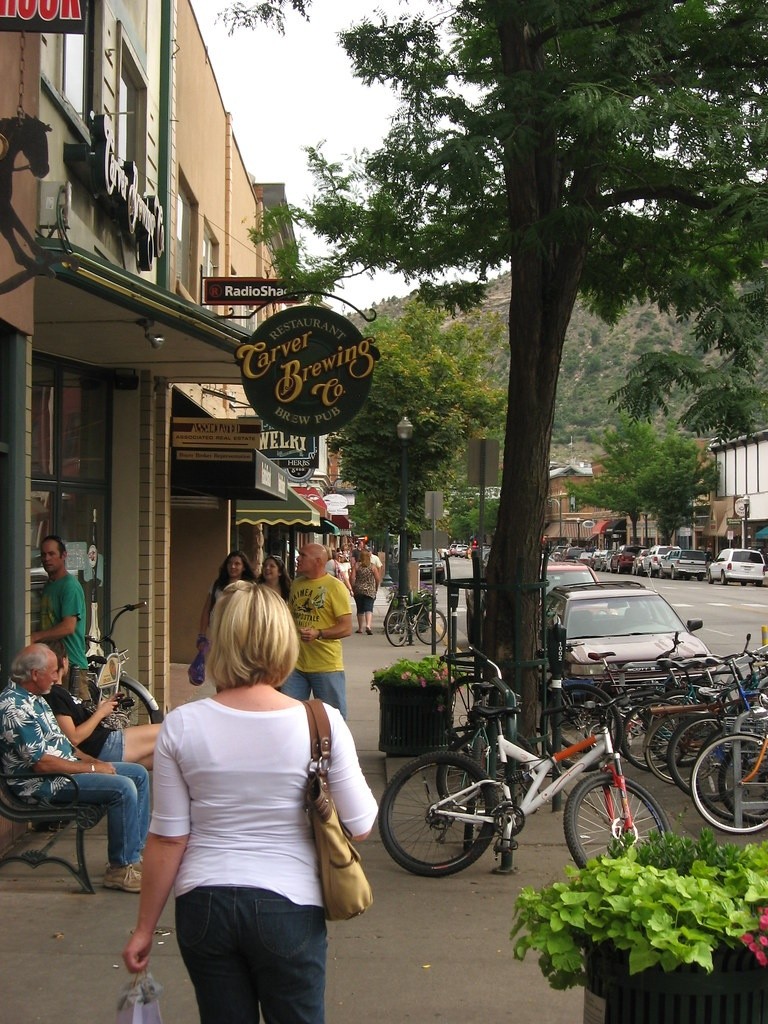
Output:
[537,580,717,716]
[412,548,445,583]
[658,549,707,582]
[539,559,618,616]
[640,544,682,577]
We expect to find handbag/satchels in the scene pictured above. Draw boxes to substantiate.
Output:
[304,698,373,923]
[334,559,344,581]
[114,966,162,1024]
[189,635,210,687]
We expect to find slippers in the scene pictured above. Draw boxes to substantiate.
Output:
[366,629,374,636]
[355,630,363,633]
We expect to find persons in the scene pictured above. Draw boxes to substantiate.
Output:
[252,553,292,604]
[323,539,383,635]
[198,549,257,695]
[32,636,161,771]
[30,535,89,703]
[288,543,352,724]
[121,582,379,1024]
[0,643,149,893]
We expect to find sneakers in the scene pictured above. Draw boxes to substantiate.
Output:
[103,863,144,892]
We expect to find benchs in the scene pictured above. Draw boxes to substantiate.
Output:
[0,746,104,895]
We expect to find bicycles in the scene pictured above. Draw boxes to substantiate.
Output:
[384,591,447,647]
[435,631,768,835]
[377,688,672,878]
[85,602,164,730]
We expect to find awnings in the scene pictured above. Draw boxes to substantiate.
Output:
[605,519,627,534]
[755,526,768,540]
[592,520,613,534]
[235,480,351,534]
[30,236,259,384]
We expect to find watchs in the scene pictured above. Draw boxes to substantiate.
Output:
[317,629,322,639]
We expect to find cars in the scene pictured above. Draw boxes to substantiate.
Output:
[707,548,766,587]
[549,546,617,572]
[438,544,490,560]
[464,552,557,645]
[607,544,646,575]
[631,548,649,577]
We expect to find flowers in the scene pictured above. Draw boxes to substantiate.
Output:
[369,655,455,713]
[507,825,768,991]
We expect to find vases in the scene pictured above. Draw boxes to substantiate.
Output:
[375,685,451,754]
[582,948,768,1024]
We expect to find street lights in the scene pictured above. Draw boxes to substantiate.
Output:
[577,517,581,547]
[743,495,750,549]
[397,416,413,609]
